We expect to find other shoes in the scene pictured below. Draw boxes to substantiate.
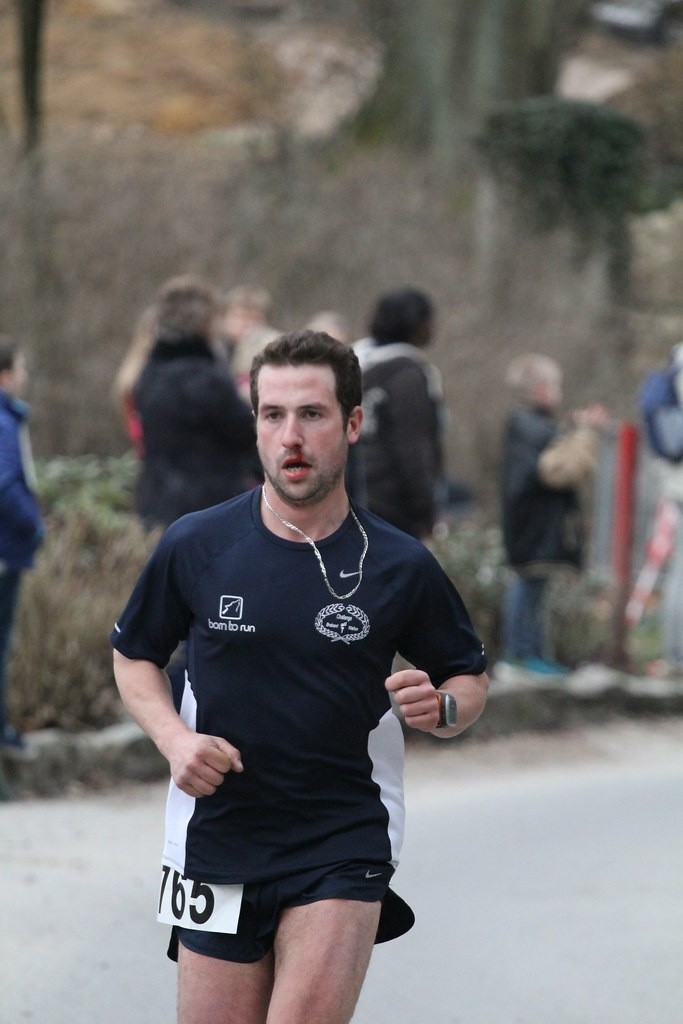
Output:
[496,653,572,688]
[0,722,39,764]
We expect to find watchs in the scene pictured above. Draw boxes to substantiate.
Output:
[435,690,458,729]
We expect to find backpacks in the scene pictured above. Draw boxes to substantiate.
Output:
[642,365,683,463]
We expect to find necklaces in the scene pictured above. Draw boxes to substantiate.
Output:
[262,485,368,598]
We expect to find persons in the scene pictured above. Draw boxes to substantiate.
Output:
[0,335,45,763]
[661,341,683,674]
[496,354,608,679]
[116,276,471,551]
[108,332,490,1024]
